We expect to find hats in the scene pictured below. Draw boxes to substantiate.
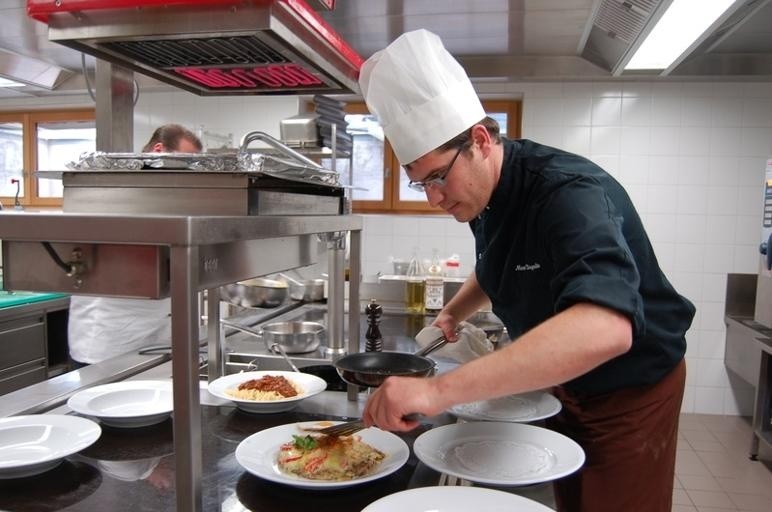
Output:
[358,27,487,166]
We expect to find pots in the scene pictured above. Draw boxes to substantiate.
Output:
[276,269,325,303]
[333,322,469,385]
[193,313,327,354]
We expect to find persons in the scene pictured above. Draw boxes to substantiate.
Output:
[359,28,696,512]
[68,125,202,370]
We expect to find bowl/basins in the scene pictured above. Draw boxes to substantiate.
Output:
[216,278,288,310]
[472,318,508,342]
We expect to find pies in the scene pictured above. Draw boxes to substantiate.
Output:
[278,435,386,481]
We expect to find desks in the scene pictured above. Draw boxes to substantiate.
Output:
[0,208,365,512]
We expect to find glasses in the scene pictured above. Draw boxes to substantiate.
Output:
[407,148,462,192]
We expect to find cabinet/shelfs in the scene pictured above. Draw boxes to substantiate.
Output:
[0,0,561,512]
[0,291,88,397]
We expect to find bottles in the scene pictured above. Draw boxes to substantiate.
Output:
[404,244,445,312]
[445,259,460,277]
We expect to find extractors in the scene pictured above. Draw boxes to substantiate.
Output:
[577,0,672,76]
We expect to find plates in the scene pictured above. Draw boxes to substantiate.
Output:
[234,419,409,487]
[1,409,103,480]
[205,413,286,443]
[356,484,557,512]
[232,472,398,511]
[447,386,558,422]
[1,464,102,510]
[66,379,177,427]
[414,422,585,486]
[208,368,328,413]
[84,422,178,464]
